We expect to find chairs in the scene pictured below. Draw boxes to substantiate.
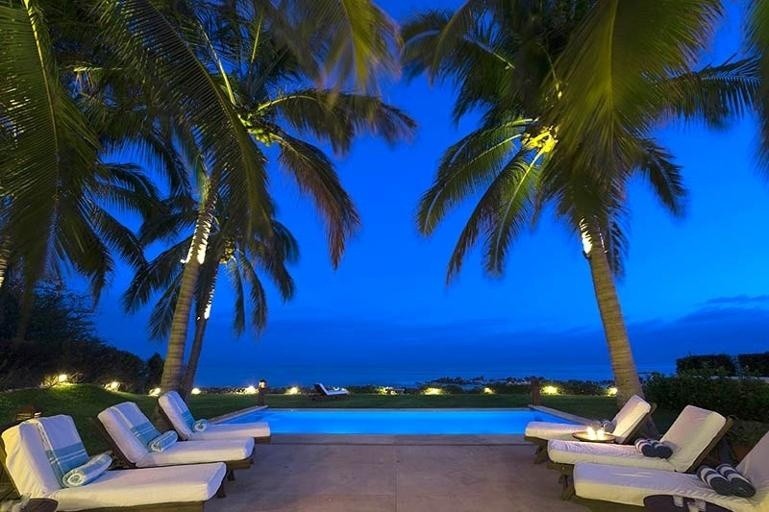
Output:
[0,389,272,511]
[522,394,769,511]
[314,382,349,400]
